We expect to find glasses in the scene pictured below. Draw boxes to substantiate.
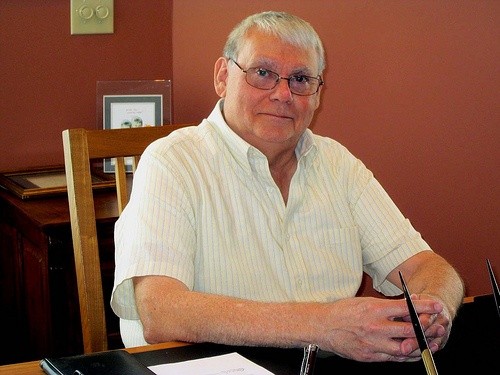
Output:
[229,56,324,96]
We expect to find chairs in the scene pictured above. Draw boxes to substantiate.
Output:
[62,125,193,355]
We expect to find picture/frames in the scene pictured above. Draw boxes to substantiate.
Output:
[102,94,163,174]
[2,169,117,200]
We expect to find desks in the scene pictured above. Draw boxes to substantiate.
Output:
[0,168,135,362]
[1,295,491,375]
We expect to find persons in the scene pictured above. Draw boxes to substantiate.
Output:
[109,10,465,363]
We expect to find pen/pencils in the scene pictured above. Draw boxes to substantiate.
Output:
[299,346,310,375]
[303,344,319,375]
